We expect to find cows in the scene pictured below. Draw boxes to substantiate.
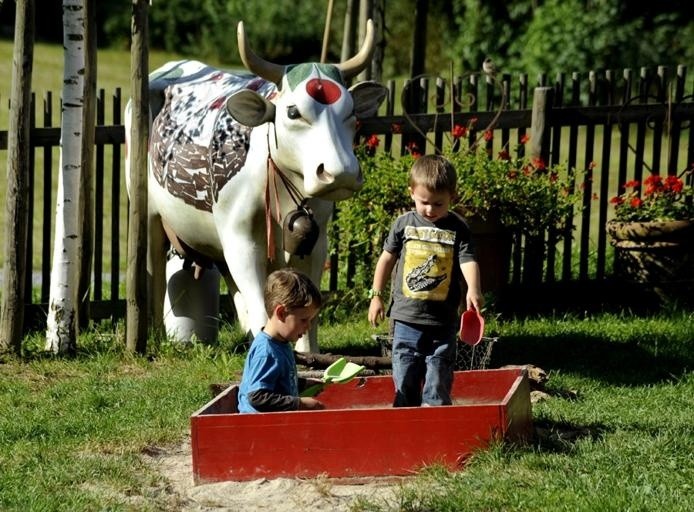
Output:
[121,17,378,353]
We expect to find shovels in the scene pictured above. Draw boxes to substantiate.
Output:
[459,304,485,346]
[300,358,366,397]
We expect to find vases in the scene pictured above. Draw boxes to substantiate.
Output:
[605,219,693,320]
[455,213,513,317]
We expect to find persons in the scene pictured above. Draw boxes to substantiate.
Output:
[237,267,325,413]
[366,156,485,406]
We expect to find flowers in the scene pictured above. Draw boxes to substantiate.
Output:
[608,173,694,224]
[326,116,598,270]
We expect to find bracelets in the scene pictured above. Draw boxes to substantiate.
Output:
[371,290,383,296]
